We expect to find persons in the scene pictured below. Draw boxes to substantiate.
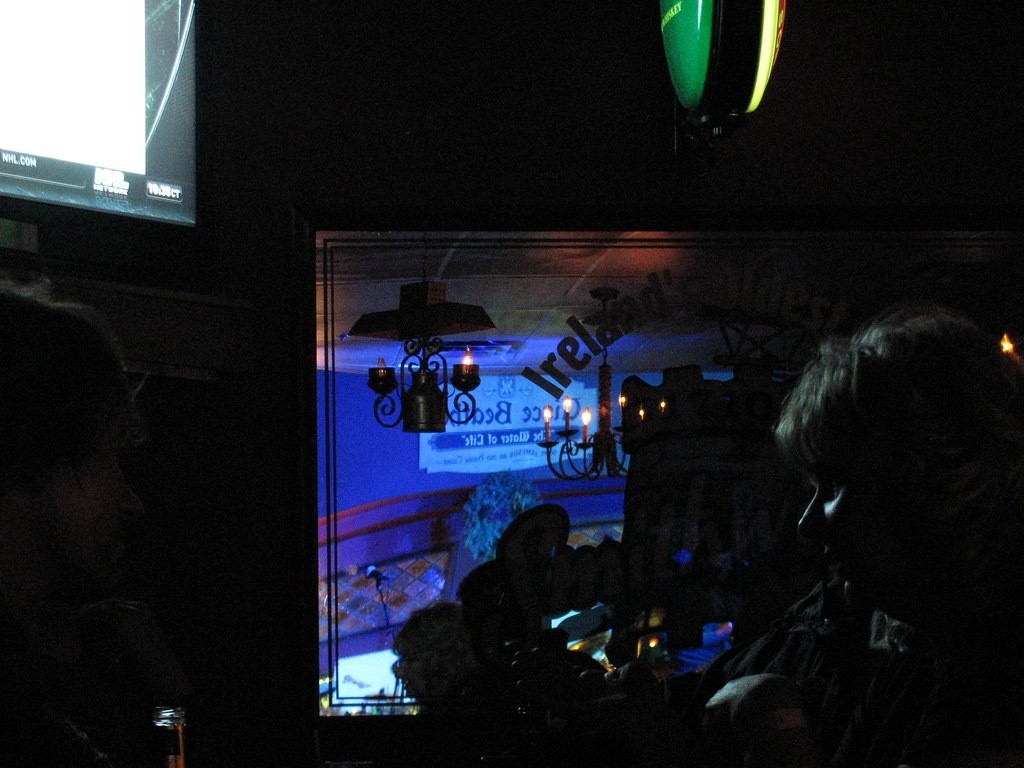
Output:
[0,293,185,768]
[388,295,1024,768]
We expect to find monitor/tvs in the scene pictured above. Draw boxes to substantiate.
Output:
[0,0,198,230]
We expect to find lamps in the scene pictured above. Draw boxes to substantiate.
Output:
[348,258,496,435]
[536,294,672,480]
